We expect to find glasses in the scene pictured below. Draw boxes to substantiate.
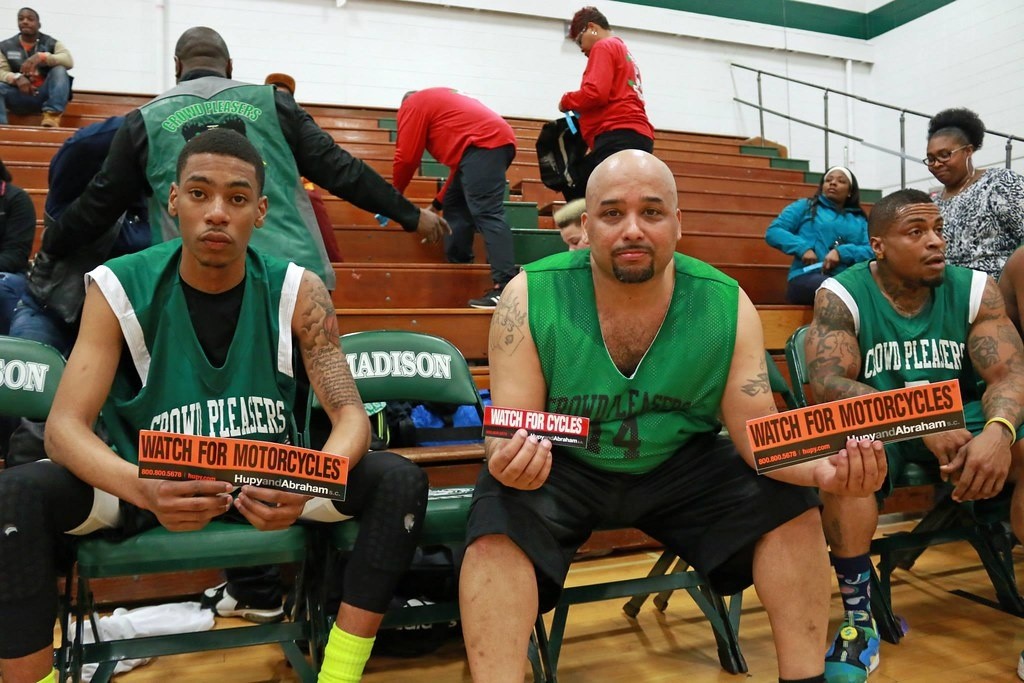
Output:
[576,26,587,46]
[923,145,967,166]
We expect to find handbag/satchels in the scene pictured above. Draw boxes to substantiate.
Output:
[535,110,587,191]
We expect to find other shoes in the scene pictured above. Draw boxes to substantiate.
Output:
[41,111,63,127]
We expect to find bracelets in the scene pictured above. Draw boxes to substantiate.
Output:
[983,417,1016,447]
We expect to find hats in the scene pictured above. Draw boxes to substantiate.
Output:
[265,73,295,94]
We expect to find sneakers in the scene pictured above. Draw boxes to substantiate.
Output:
[468,289,502,309]
[201,580,285,623]
[825,610,880,683]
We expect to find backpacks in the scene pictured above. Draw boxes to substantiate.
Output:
[326,544,463,657]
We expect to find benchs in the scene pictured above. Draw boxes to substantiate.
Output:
[0,91,937,555]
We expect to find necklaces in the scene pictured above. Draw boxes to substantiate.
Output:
[941,175,974,205]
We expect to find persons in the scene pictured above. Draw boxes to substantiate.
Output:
[264,73,340,262]
[559,8,654,203]
[923,110,1024,285]
[392,88,522,310]
[765,166,881,306]
[0,130,430,683]
[0,110,154,466]
[0,7,73,127]
[41,26,452,625]
[458,149,888,683]
[805,189,1024,683]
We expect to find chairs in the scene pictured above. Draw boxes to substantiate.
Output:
[0,322,1024,683]
